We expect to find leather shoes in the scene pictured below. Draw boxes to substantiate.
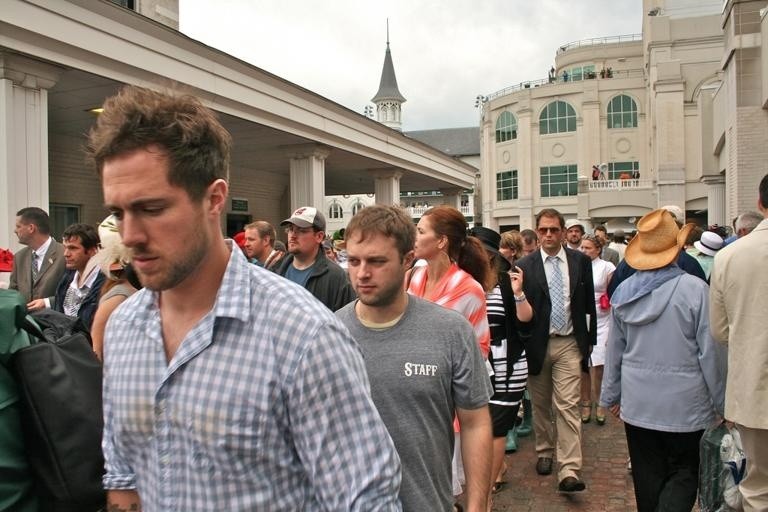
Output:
[558,478,584,491]
[536,458,552,475]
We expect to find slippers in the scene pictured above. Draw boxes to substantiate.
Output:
[491,462,506,494]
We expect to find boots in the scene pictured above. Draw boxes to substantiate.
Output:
[515,399,532,437]
[504,430,517,450]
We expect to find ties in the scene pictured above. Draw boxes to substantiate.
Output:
[546,256,565,331]
[30,253,38,283]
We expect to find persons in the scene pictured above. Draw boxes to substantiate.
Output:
[550,66,557,78]
[586,66,613,79]
[233,205,628,511]
[591,164,601,179]
[82,84,420,511]
[0,206,144,511]
[563,70,569,81]
[600,176,767,512]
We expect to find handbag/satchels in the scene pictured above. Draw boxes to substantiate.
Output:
[10,333,107,503]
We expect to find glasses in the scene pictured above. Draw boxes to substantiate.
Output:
[284,227,314,233]
[537,228,562,235]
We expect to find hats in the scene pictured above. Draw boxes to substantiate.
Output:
[693,231,723,257]
[471,226,510,271]
[79,214,129,283]
[279,207,325,232]
[563,219,583,235]
[624,209,695,270]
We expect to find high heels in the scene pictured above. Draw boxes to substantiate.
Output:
[581,403,591,423]
[594,401,605,423]
